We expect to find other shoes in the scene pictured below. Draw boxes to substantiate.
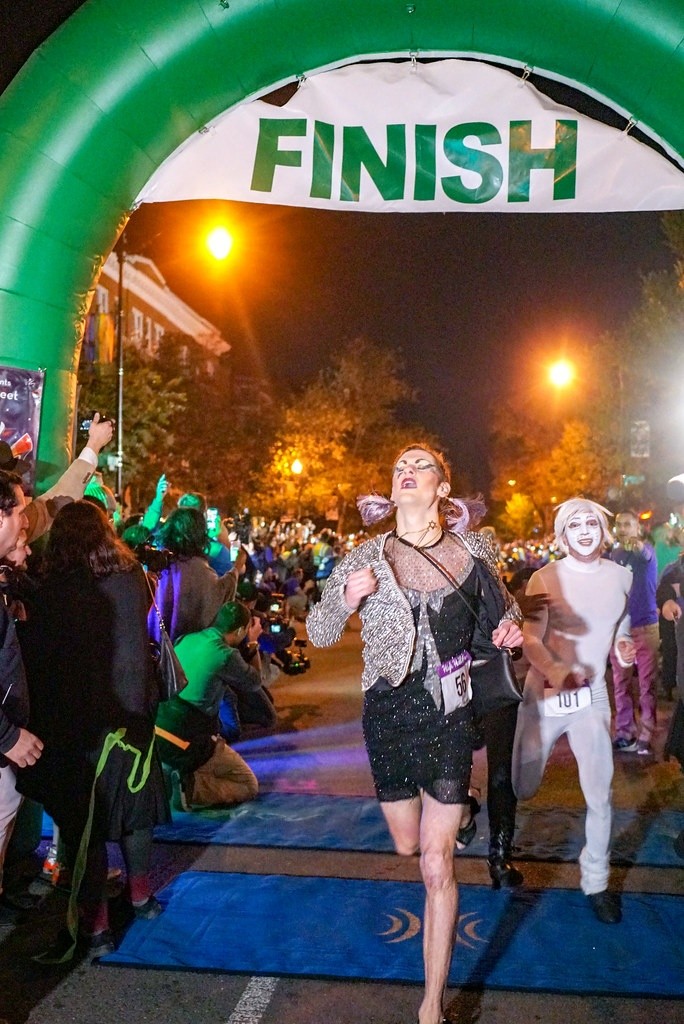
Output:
[485,857,524,888]
[42,856,61,874]
[179,773,194,812]
[51,862,121,898]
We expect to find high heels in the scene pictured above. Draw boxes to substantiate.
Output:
[456,795,481,850]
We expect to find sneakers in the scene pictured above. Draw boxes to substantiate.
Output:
[128,859,176,907]
[612,737,639,752]
[636,739,653,755]
[588,890,621,924]
[75,897,110,930]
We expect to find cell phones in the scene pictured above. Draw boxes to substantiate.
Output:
[206,508,218,530]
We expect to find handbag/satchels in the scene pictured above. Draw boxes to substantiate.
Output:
[459,646,525,730]
[146,628,188,700]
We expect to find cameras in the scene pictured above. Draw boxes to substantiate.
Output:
[269,601,281,614]
[231,540,241,562]
[270,623,287,634]
[80,416,107,439]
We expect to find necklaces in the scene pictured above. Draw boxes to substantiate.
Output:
[396,520,442,548]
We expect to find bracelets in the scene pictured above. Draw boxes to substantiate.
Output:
[247,640,258,648]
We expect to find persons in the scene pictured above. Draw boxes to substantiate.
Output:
[306,446,524,1024]
[456,474,684,923]
[0,412,371,958]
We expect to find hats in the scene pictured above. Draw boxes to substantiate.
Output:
[0,440,31,476]
